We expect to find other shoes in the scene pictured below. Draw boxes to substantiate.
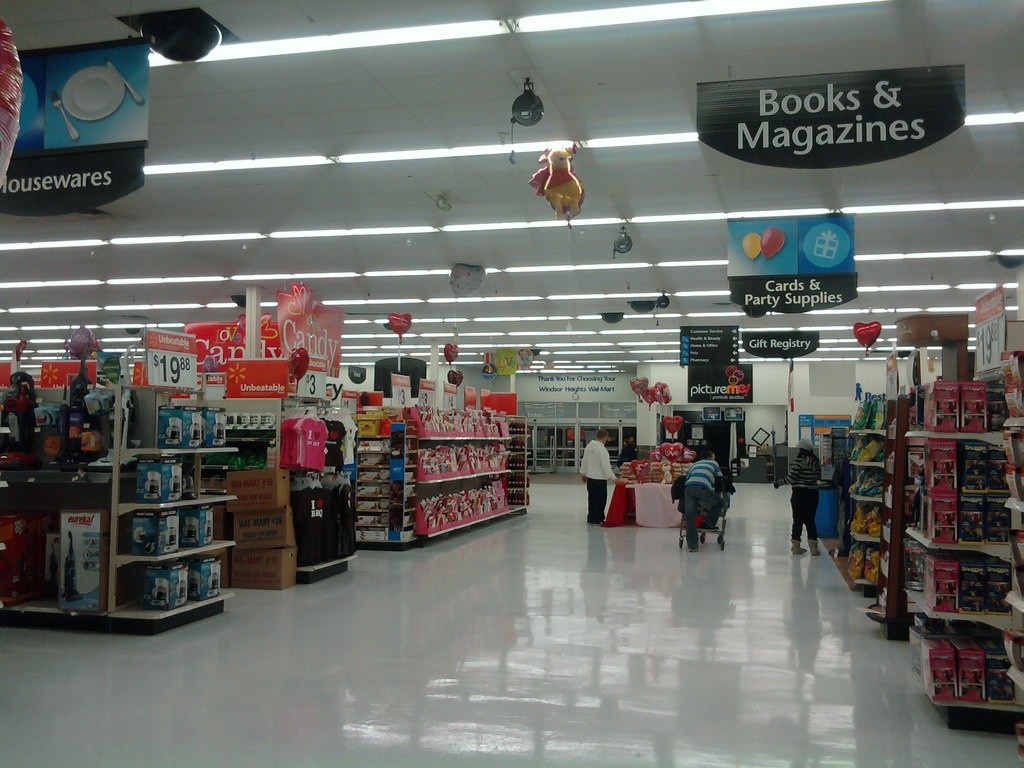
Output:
[589,520,605,526]
[689,548,696,552]
[701,522,719,532]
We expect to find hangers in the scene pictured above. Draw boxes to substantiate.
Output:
[297,473,351,494]
[291,404,338,422]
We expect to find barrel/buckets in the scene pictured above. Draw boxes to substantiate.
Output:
[812,484,840,540]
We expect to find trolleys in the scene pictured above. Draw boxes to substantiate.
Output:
[678,475,731,552]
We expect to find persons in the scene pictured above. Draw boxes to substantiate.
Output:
[774,438,822,556]
[579,429,618,525]
[685,451,724,551]
[617,437,636,468]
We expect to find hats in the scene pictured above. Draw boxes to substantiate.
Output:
[795,438,814,450]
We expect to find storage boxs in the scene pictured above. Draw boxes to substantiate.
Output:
[0,511,52,607]
[916,379,1017,704]
[132,409,299,612]
[58,508,137,612]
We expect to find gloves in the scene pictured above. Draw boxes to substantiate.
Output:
[774,482,780,489]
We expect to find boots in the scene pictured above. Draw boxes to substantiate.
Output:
[807,539,821,556]
[790,538,807,554]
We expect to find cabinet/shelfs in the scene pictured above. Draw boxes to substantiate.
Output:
[0,388,127,631]
[847,428,887,598]
[347,415,540,550]
[108,386,240,635]
[903,351,1024,720]
